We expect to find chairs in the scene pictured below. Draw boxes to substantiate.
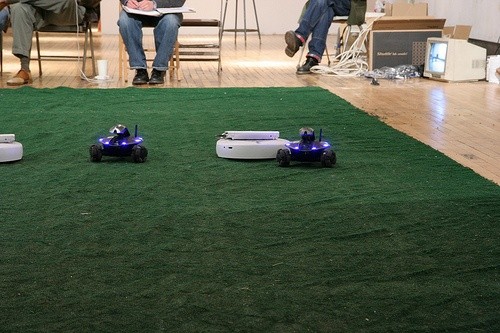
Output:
[29,0,96,80]
[296,15,368,68]
[118,0,223,83]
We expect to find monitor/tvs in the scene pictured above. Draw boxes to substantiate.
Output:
[423,38,487,85]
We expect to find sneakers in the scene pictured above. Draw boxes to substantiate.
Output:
[7,68,32,84]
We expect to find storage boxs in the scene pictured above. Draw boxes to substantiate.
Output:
[337,0,472,72]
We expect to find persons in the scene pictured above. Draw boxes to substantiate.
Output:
[285,0,367,74]
[117,0,187,83]
[0,0,102,85]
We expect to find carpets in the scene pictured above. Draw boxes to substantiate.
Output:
[1,86,500,332]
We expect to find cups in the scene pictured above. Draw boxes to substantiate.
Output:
[97,60,108,79]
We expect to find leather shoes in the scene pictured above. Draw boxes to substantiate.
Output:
[150,69,165,83]
[297,58,319,73]
[132,69,149,84]
[285,30,300,57]
